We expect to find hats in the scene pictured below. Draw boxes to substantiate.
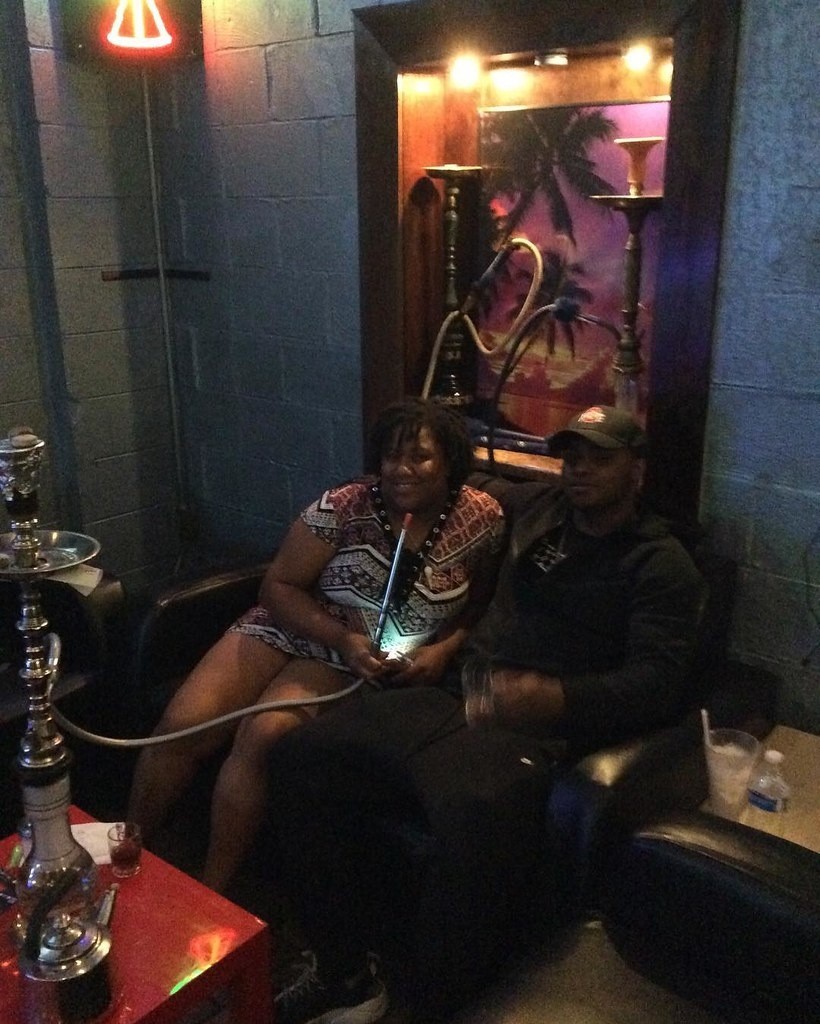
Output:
[547,405,651,459]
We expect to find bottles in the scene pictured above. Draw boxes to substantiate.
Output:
[744,749,790,839]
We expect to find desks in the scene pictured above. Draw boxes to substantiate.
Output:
[602,713,820,1024]
[0,804,275,1024]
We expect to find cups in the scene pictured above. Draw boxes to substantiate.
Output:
[704,728,760,821]
[108,823,143,878]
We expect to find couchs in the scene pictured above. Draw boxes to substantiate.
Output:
[0,574,132,835]
[137,469,782,948]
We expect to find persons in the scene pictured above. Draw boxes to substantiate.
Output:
[127,396,508,892]
[270,403,723,1024]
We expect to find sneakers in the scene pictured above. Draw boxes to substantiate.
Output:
[271,950,390,1023]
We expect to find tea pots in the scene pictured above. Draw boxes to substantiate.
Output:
[17,884,115,1024]
